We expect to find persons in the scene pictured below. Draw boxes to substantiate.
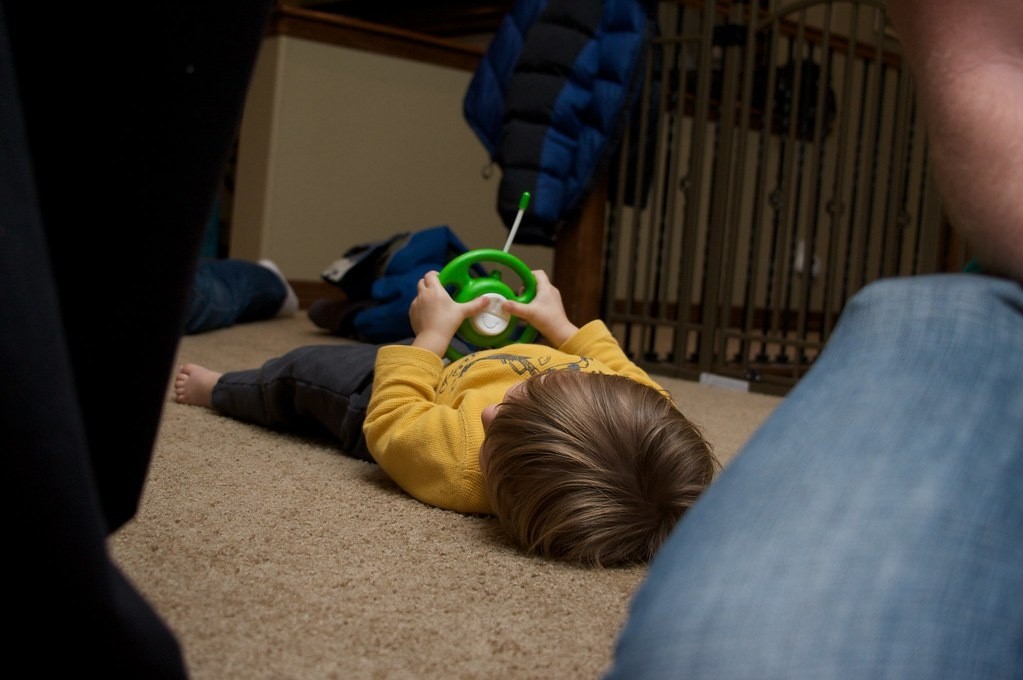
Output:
[174,269,717,566]
[611,0,1023,680]
[181,257,299,336]
